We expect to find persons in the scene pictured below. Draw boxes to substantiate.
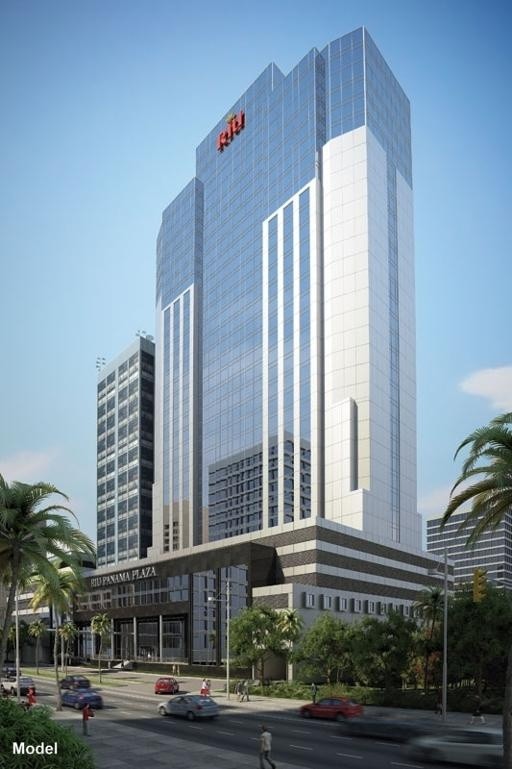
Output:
[259,725,276,769]
[121,659,124,669]
[26,687,36,704]
[172,663,177,677]
[468,695,486,725]
[82,703,92,737]
[311,682,317,704]
[178,664,180,677]
[201,679,211,698]
[234,678,250,702]
